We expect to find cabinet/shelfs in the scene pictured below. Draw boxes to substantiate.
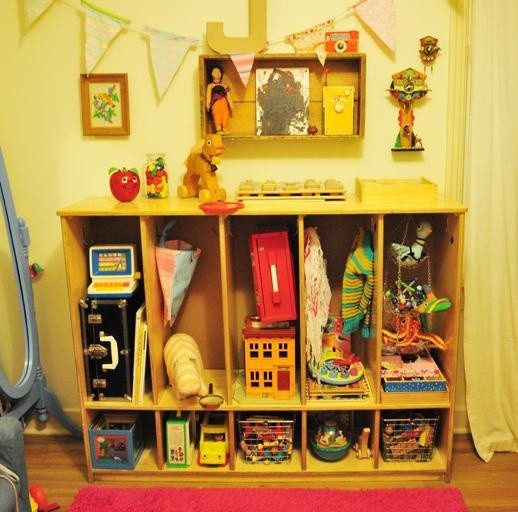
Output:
[55,195,468,483]
[198,52,366,142]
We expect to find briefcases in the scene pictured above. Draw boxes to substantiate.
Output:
[79,289,144,401]
[250,229,298,324]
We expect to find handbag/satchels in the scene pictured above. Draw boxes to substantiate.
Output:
[157,220,206,328]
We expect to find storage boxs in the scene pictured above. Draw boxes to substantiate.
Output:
[88,412,145,470]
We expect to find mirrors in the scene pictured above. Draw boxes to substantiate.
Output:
[0,146,83,441]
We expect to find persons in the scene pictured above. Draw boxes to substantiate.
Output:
[206,65,234,137]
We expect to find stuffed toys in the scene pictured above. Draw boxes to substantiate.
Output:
[163,332,209,402]
[108,167,141,201]
[388,222,433,268]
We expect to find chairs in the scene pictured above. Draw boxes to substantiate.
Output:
[0,416,32,512]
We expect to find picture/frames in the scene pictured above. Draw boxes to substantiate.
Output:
[80,72,130,137]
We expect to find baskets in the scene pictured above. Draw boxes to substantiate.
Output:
[380,412,440,463]
[236,414,296,465]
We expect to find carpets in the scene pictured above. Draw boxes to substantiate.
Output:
[66,485,468,512]
